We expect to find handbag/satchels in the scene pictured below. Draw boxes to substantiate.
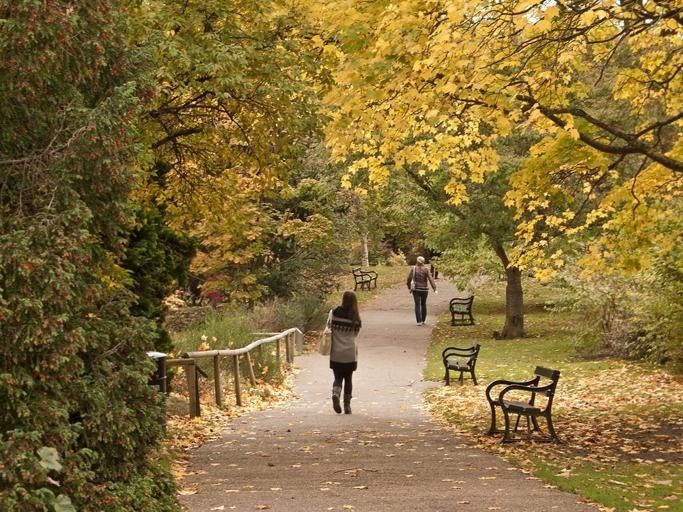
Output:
[319,309,333,356]
[410,266,416,291]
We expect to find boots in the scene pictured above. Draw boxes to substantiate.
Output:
[344,393,352,415]
[332,385,342,414]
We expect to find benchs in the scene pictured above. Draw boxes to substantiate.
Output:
[485,365,560,444]
[449,296,476,325]
[352,267,378,291]
[441,343,481,386]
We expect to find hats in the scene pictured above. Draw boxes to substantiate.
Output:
[417,256,425,263]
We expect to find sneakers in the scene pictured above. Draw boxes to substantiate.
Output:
[417,320,426,326]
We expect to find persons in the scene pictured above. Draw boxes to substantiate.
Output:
[405,256,438,326]
[427,246,442,279]
[325,289,361,414]
